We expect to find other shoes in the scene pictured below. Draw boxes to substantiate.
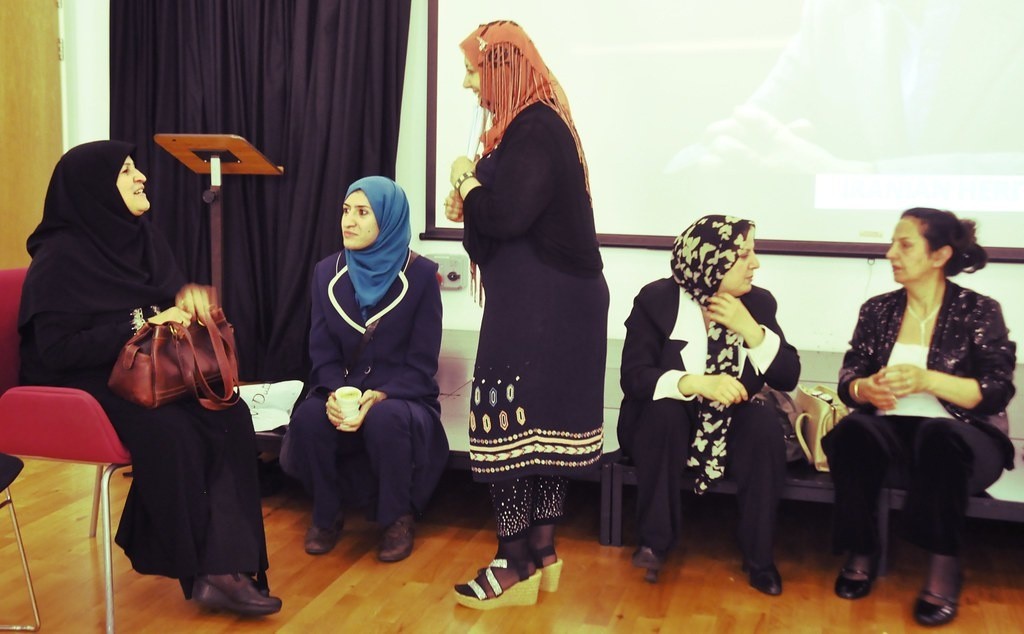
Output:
[834,554,877,600]
[912,572,961,625]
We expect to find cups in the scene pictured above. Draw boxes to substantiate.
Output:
[335,387,362,423]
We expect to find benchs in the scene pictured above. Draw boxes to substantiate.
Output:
[246,328,1024,575]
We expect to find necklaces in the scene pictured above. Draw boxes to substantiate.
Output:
[907,304,941,344]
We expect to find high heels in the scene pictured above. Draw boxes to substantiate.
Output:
[743,549,782,594]
[304,508,345,555]
[453,552,542,609]
[192,572,282,615]
[632,545,668,582]
[477,545,562,592]
[378,514,414,562]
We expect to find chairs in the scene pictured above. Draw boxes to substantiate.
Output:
[0,267,130,634]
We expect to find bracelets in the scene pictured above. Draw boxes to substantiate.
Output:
[854,380,861,396]
[454,172,475,191]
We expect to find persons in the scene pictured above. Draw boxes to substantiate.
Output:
[616,214,802,595]
[820,204,1016,626]
[19,138,282,617]
[280,176,450,561]
[445,20,609,611]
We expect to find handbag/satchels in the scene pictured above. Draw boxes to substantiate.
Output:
[794,384,848,472]
[763,384,804,465]
[108,304,240,410]
